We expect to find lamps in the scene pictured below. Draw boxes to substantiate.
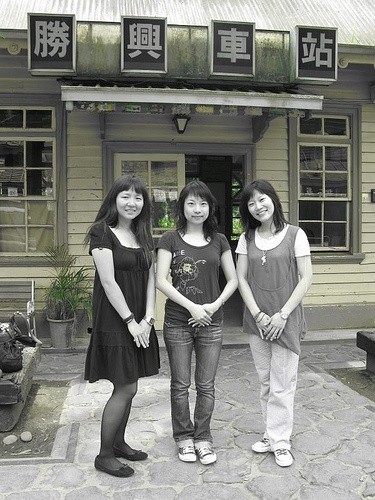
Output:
[172,114,192,134]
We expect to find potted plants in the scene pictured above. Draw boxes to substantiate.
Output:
[39,244,91,353]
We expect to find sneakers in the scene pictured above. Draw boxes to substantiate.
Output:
[198,447,216,464]
[252,438,271,453]
[274,449,294,467]
[179,446,197,461]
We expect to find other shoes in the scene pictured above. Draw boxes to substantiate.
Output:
[95,455,133,476]
[113,449,148,461]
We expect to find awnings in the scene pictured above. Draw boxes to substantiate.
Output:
[60,80,324,119]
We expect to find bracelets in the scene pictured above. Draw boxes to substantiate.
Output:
[122,313,134,325]
[253,311,267,323]
[218,297,225,306]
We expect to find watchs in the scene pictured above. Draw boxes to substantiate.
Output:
[142,315,156,326]
[278,310,289,320]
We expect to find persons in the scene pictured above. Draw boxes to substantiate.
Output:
[82,176,161,478]
[234,180,313,468]
[156,179,238,465]
